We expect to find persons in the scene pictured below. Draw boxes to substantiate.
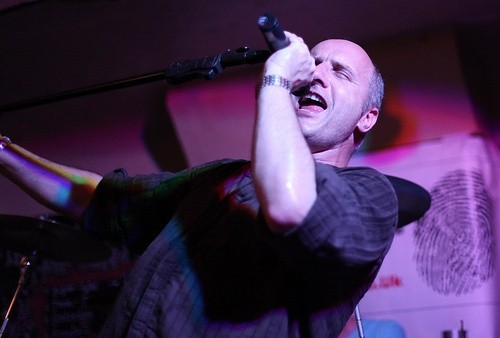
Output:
[0,31,397,337]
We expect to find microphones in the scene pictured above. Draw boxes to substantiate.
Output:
[259,13,291,53]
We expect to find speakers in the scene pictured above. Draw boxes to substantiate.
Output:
[458,16,499,132]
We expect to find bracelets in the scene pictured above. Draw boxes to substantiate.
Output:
[260,74,293,91]
